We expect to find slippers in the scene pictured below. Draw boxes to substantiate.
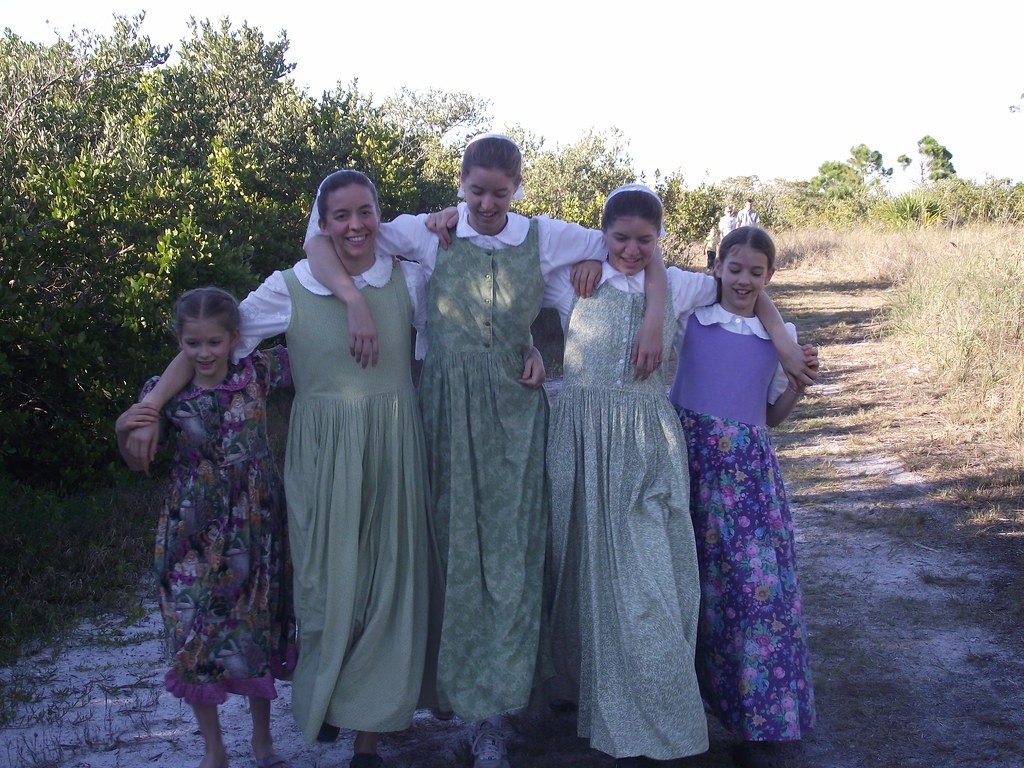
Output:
[251,741,291,768]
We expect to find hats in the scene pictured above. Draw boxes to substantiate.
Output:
[745,199,753,202]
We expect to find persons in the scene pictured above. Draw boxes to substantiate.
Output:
[703,199,761,273]
[570,226,819,741]
[424,184,819,768]
[115,255,294,768]
[305,138,667,768]
[126,171,546,768]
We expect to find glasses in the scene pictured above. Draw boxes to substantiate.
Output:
[727,211,732,213]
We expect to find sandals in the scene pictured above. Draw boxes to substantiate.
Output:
[735,735,786,768]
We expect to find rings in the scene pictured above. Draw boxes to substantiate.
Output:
[655,360,662,364]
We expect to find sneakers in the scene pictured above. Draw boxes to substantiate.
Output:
[430,690,454,721]
[469,714,511,768]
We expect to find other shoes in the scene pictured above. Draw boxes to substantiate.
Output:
[706,268,710,272]
[350,753,384,768]
[317,721,340,743]
